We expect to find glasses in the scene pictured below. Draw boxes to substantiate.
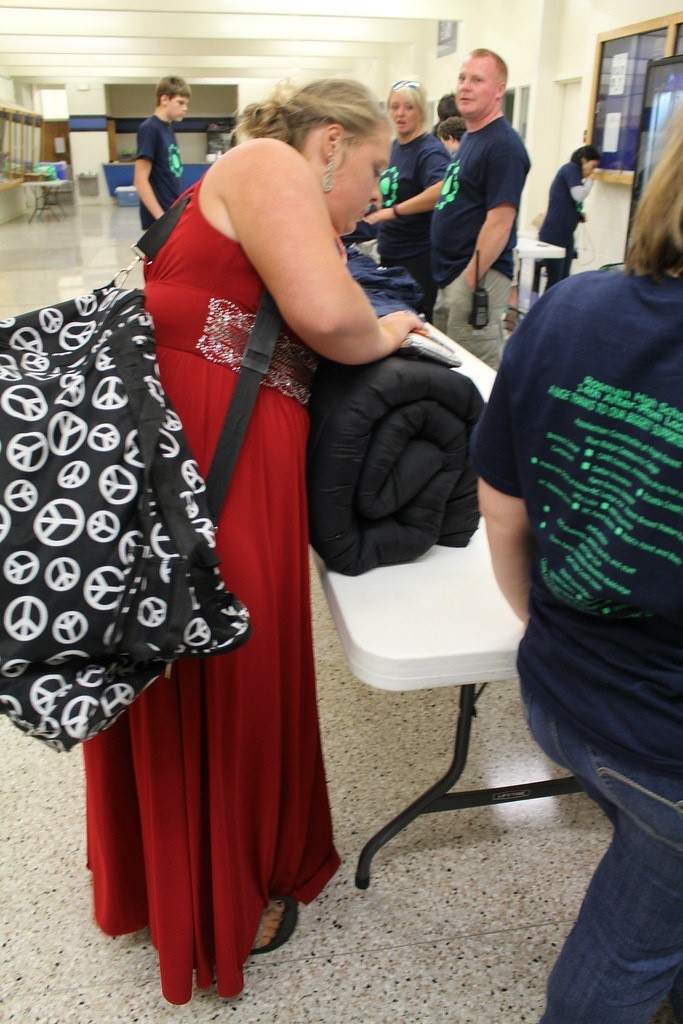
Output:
[392,81,421,90]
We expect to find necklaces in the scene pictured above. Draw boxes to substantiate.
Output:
[332,229,349,266]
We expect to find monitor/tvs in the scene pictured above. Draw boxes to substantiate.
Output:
[626,54,683,238]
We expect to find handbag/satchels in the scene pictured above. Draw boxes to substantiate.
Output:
[0,277,251,752]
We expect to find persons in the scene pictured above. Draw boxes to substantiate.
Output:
[359,80,453,325]
[538,144,601,294]
[83,78,428,1006]
[462,99,683,1023]
[132,74,189,229]
[427,48,532,372]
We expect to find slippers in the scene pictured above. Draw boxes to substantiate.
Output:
[249,894,298,955]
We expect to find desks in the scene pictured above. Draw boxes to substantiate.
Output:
[311,321,583,893]
[516,237,569,321]
[22,179,69,224]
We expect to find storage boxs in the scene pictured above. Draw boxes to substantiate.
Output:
[115,186,141,208]
[37,161,67,180]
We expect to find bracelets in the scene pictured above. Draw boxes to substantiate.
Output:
[392,203,401,219]
